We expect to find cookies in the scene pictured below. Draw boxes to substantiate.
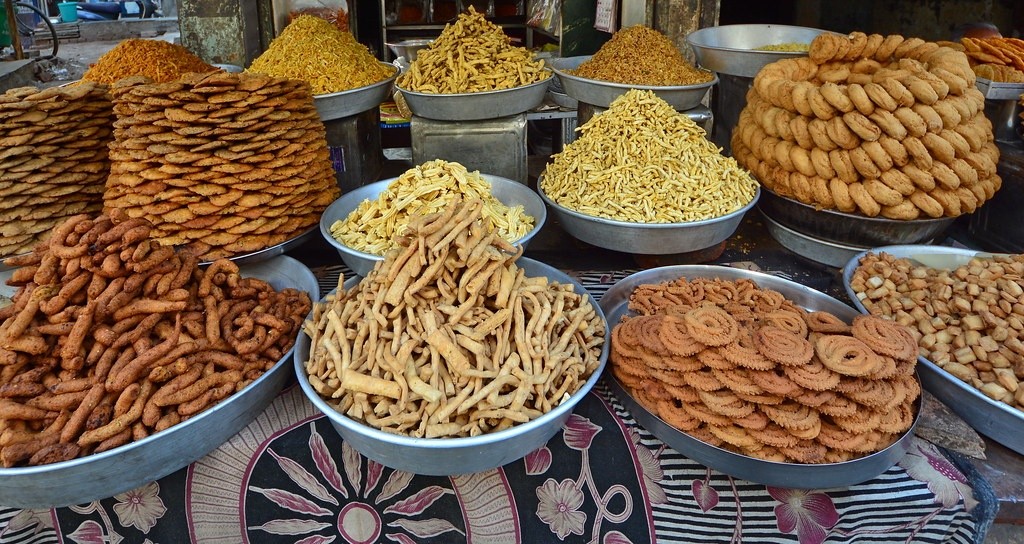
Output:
[0,68,340,260]
[729,29,1002,221]
[611,276,920,466]
[935,36,1024,83]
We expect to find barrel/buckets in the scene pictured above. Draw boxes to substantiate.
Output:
[0,0,19,47]
[57,2,78,23]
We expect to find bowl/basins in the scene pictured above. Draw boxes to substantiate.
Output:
[194,225,321,270]
[313,61,401,121]
[1,254,321,507]
[385,36,437,62]
[293,256,611,477]
[395,65,555,121]
[598,263,924,488]
[58,64,245,89]
[537,163,761,255]
[843,244,1024,455]
[686,23,844,78]
[756,185,971,269]
[550,54,719,110]
[318,173,547,278]
